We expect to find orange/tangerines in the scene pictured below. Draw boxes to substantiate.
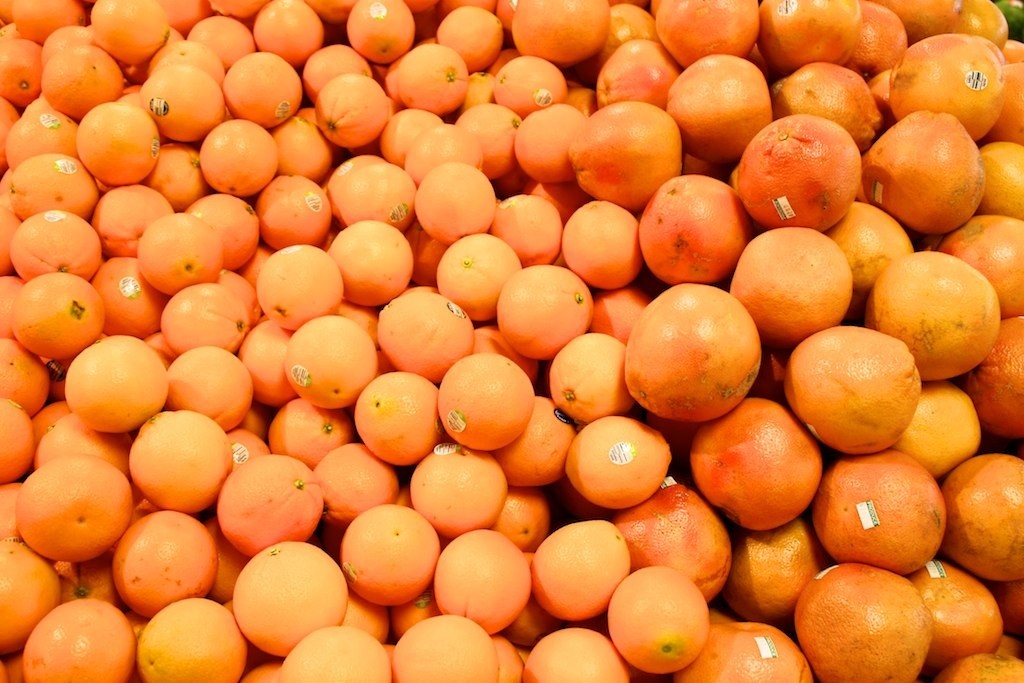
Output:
[2,0,1023,683]
